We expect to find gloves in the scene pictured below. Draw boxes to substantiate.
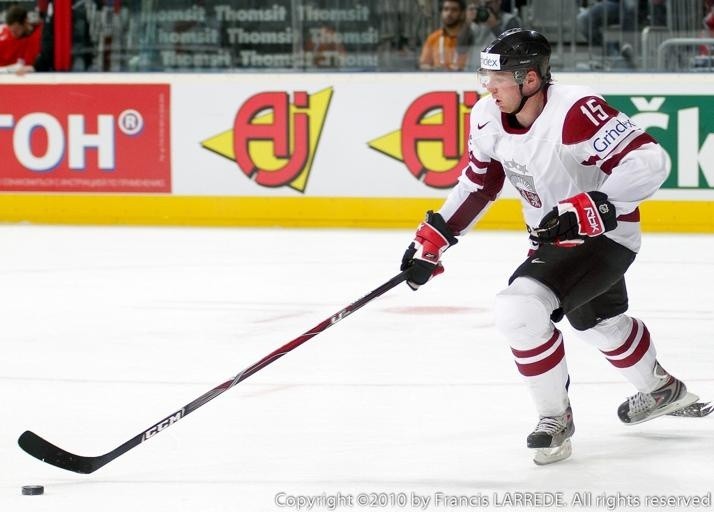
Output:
[400,209,457,291]
[535,191,618,247]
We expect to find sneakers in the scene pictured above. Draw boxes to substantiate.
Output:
[618,377,686,423]
[528,405,575,449]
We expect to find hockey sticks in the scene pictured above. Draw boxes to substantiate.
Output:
[18,271,410,474]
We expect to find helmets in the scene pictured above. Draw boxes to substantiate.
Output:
[477,27,551,88]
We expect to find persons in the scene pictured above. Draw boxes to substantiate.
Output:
[418,0,472,70]
[457,0,521,72]
[0,5,45,68]
[576,0,667,69]
[399,25,686,449]
